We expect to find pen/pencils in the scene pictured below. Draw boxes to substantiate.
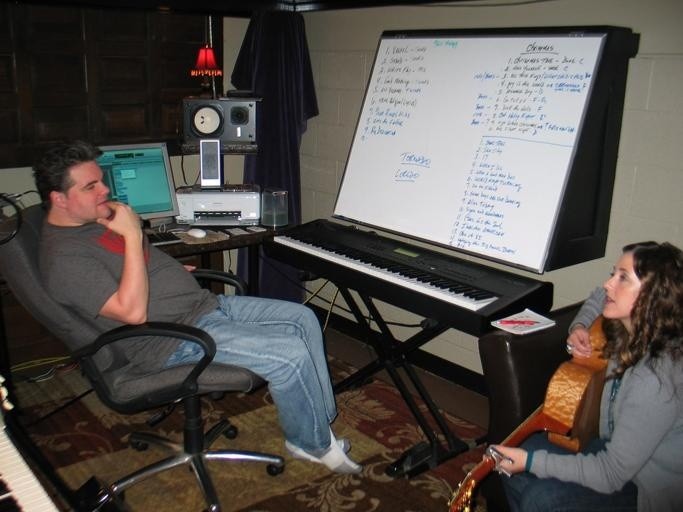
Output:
[498,319,540,325]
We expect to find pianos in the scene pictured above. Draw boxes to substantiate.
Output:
[0,392,77,512]
[262,218,553,337]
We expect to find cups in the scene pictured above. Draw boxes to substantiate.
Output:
[261,190,288,228]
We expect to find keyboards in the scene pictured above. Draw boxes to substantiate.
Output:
[145,230,185,248]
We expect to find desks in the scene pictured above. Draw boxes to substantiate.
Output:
[139,220,267,294]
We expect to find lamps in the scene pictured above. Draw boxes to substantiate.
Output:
[190,45,222,99]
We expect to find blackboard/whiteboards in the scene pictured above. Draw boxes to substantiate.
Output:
[332,33,608,275]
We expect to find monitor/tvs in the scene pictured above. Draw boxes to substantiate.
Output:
[96,143,180,220]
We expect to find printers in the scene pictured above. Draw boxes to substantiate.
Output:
[174,184,262,227]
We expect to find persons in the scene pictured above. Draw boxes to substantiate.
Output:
[32,138,365,474]
[483,241,681,511]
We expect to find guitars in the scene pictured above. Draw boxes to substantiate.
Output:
[446,315,607,512]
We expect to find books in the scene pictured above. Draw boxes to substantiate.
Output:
[491,307,556,335]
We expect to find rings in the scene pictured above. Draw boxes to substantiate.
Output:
[566,342,573,352]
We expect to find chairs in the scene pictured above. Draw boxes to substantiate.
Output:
[0,204,285,512]
[479,299,584,512]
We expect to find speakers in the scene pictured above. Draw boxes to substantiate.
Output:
[177,97,263,145]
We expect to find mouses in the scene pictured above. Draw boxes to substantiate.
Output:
[188,228,208,239]
